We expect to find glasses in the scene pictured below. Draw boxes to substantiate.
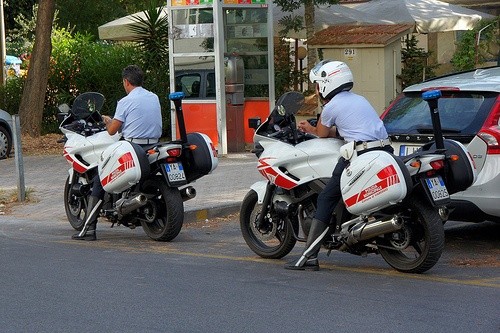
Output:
[121,81,125,86]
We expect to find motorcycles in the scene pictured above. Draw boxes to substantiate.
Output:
[57,92,219,242]
[238,91,451,274]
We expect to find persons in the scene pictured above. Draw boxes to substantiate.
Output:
[286,59,394,270]
[72,65,162,242]
[5,68,18,80]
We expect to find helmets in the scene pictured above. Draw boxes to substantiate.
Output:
[309,61,354,100]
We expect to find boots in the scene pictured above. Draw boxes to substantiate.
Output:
[284,218,330,271]
[72,195,104,241]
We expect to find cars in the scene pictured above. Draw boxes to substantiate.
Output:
[378,67,500,224]
[0,109,13,160]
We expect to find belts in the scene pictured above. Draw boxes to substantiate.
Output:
[124,137,158,145]
[356,139,390,152]
